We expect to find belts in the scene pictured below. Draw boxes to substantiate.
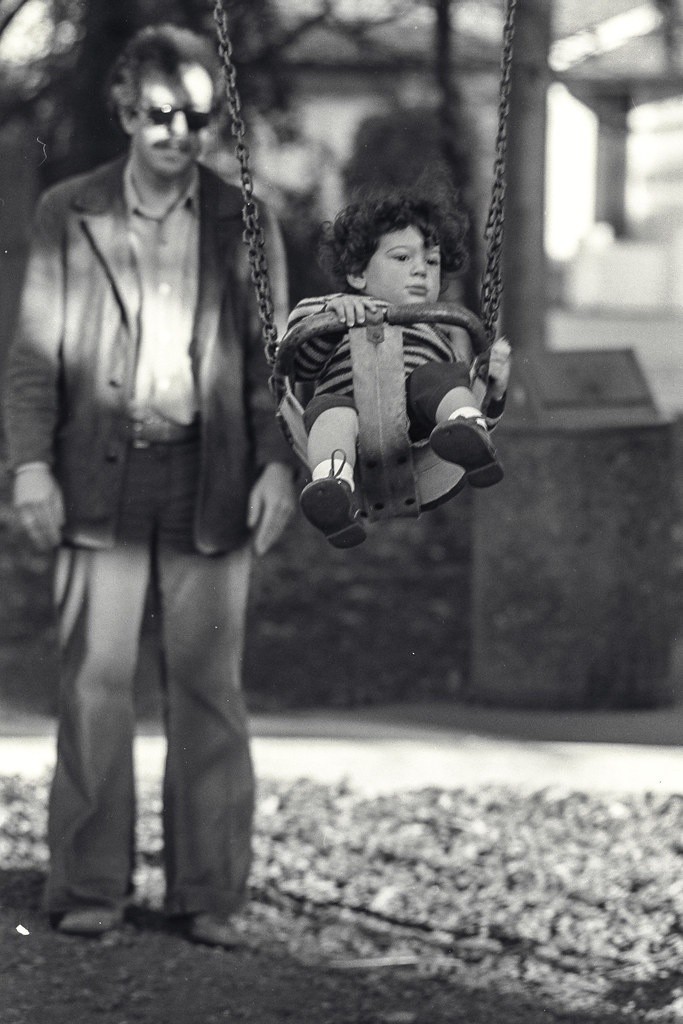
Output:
[130,418,200,447]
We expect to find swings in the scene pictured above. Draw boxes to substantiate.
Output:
[214,0,520,523]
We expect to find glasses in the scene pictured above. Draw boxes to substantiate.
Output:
[136,106,210,129]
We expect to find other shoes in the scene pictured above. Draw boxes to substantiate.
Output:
[430,415,505,488]
[301,478,367,549]
[58,906,123,931]
[164,899,248,947]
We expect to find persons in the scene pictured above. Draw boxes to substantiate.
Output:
[288,167,511,550]
[4,25,304,951]
